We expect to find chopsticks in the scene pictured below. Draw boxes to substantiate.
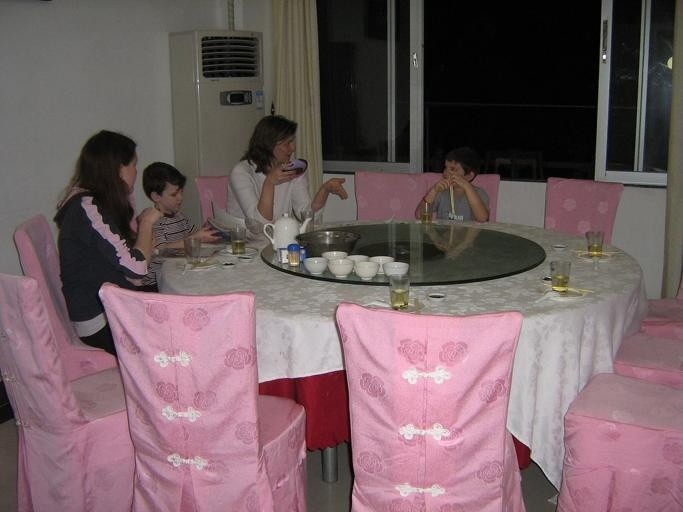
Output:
[448,174,456,218]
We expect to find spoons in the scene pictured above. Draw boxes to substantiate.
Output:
[361,299,390,307]
[592,256,601,271]
[535,291,560,303]
[182,262,195,276]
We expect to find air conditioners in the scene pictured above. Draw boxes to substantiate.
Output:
[167,27,267,229]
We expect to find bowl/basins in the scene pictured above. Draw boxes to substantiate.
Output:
[304,250,409,280]
[296,228,360,257]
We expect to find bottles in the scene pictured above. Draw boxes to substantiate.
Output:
[287,244,305,267]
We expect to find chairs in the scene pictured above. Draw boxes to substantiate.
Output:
[543,177,623,242]
[614,329,682,388]
[332,302,522,508]
[0,273,135,507]
[422,172,504,223]
[642,287,682,333]
[556,375,682,507]
[98,283,308,507]
[14,213,119,381]
[353,167,424,219]
[194,174,229,229]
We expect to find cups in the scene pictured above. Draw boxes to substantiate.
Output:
[184,238,200,264]
[551,261,571,294]
[586,231,604,258]
[300,211,314,232]
[231,229,246,254]
[389,274,410,310]
[421,203,432,224]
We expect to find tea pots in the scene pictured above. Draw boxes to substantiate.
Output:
[264,212,313,251]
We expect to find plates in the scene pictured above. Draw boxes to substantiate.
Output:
[193,257,220,269]
[546,287,584,302]
[577,253,610,261]
[392,300,425,312]
[219,248,258,257]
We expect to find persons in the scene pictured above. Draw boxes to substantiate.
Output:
[415,147,490,223]
[227,115,348,232]
[136,161,223,289]
[54,128,157,355]
[423,223,482,259]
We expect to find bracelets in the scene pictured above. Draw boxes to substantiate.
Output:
[423,197,432,207]
